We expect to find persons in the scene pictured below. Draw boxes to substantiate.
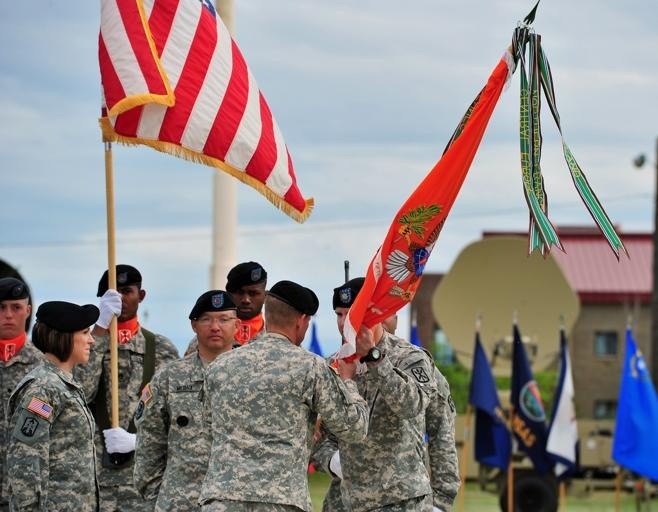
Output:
[194,279,371,512]
[131,288,237,512]
[183,261,269,356]
[306,309,463,512]
[0,300,102,512]
[318,275,441,512]
[70,264,181,512]
[0,277,46,512]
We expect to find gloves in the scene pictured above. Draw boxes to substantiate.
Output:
[328,450,344,479]
[95,290,122,329]
[432,505,443,512]
[102,427,137,453]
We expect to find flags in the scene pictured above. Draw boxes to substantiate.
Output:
[306,319,326,358]
[506,315,551,482]
[94,0,315,225]
[331,0,632,366]
[408,310,432,441]
[457,313,516,476]
[537,313,581,486]
[611,313,657,486]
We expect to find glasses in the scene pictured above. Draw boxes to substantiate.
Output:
[195,315,236,325]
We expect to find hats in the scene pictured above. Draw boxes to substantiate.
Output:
[267,279,319,317]
[188,289,238,321]
[96,264,143,297]
[0,277,29,303]
[35,301,100,333]
[226,261,268,287]
[332,276,367,310]
